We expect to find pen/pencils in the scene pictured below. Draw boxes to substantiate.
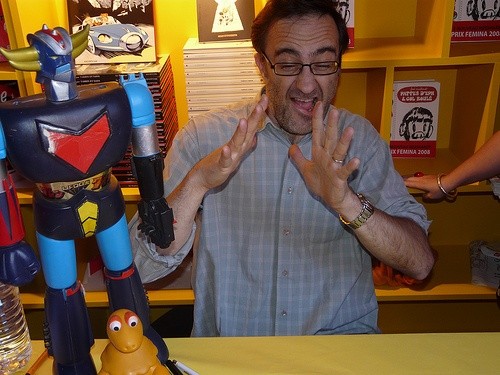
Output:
[173,360,199,375]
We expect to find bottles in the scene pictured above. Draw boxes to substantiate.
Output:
[0,283,32,375]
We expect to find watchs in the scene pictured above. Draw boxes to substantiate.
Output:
[338,192,375,230]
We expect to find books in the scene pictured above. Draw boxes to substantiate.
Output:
[451,0,500,43]
[66,0,179,188]
[183,0,266,120]
[335,0,354,49]
[390,79,440,160]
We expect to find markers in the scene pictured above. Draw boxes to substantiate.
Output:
[166,360,183,375]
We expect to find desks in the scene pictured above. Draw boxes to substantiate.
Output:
[0,331,500,375]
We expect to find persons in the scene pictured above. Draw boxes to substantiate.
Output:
[404,129,500,203]
[126,0,433,339]
[0,23,176,375]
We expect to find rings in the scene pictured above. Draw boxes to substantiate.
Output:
[329,154,345,167]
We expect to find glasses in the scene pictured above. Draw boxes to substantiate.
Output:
[261,47,342,76]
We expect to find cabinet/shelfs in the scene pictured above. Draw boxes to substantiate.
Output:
[252,0,500,303]
[0,0,196,308]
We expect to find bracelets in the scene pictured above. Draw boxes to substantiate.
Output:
[437,173,457,197]
